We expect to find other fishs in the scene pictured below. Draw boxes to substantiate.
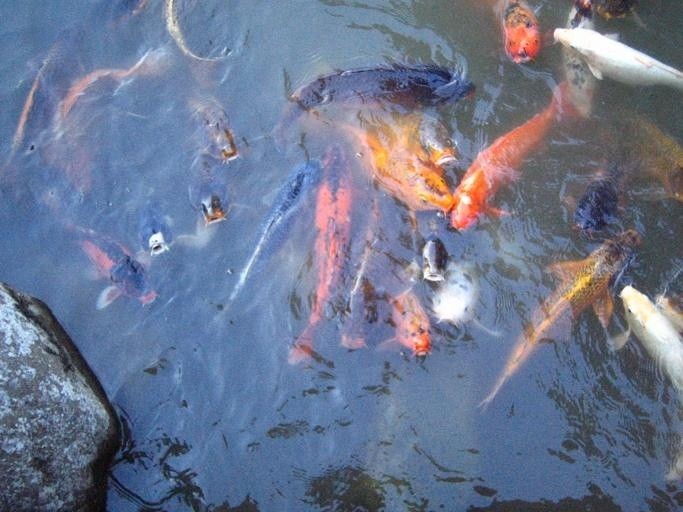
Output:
[0,1,683,417]
